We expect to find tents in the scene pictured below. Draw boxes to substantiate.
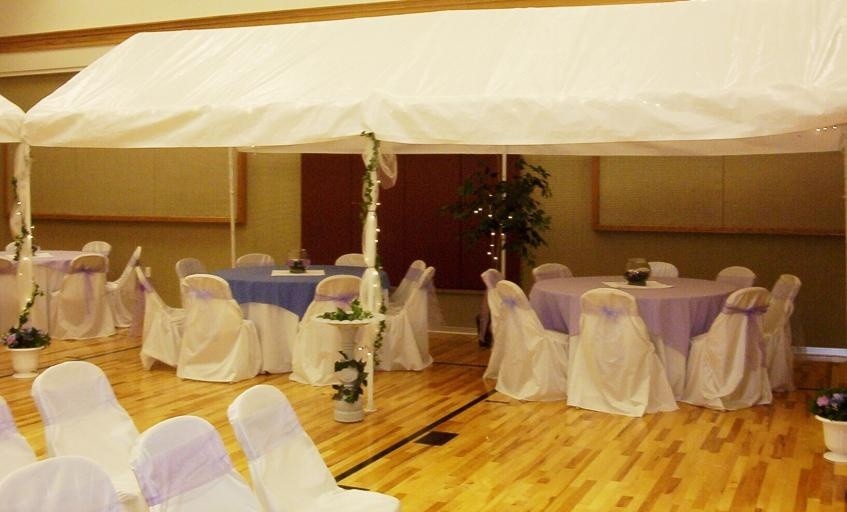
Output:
[0,91,24,257]
[20,1,845,414]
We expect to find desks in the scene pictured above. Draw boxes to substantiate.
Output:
[533,277,732,384]
[2,250,86,337]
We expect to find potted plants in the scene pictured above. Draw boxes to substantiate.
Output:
[311,299,384,423]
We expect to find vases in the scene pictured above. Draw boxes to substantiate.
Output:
[8,348,44,379]
[435,156,551,348]
[813,415,847,462]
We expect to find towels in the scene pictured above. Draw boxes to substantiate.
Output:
[602,279,672,289]
[271,269,326,276]
[7,252,51,259]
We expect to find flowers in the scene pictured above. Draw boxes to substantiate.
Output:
[808,387,847,421]
[5,285,52,348]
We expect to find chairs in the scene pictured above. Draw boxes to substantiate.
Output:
[0,258,14,334]
[82,241,111,257]
[32,362,141,512]
[769,274,800,391]
[481,268,503,307]
[209,264,385,368]
[335,253,367,267]
[226,385,399,512]
[495,282,571,401]
[137,266,185,369]
[289,275,367,385]
[132,416,265,512]
[103,246,142,323]
[7,241,19,253]
[648,262,678,278]
[687,288,769,410]
[566,289,679,418]
[180,274,261,383]
[1,456,125,512]
[0,397,36,481]
[532,264,573,278]
[236,253,274,266]
[51,255,108,337]
[718,269,756,288]
[384,260,425,315]
[175,258,204,283]
[378,266,435,370]
